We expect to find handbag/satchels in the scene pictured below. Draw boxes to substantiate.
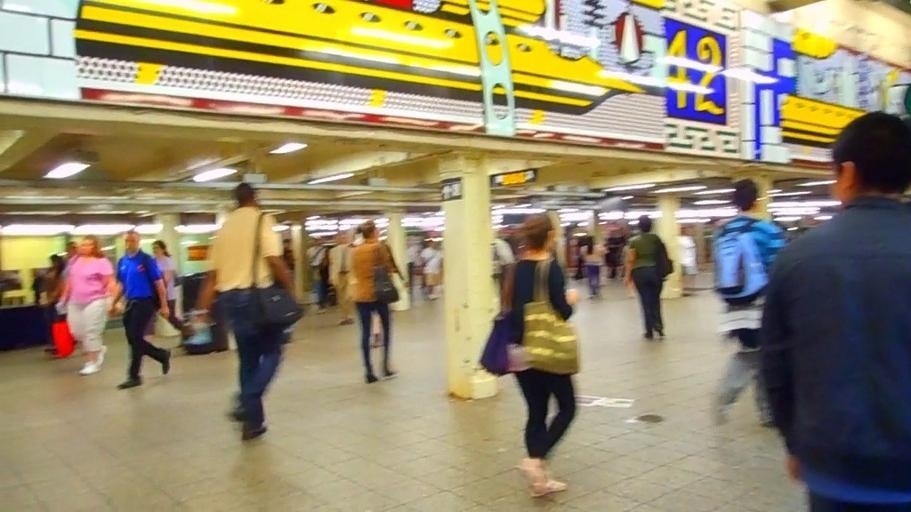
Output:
[521,256,579,375]
[374,243,400,304]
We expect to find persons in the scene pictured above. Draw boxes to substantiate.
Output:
[145,242,193,341]
[58,235,123,375]
[419,239,445,298]
[195,183,298,440]
[307,239,325,298]
[498,214,579,496]
[759,111,909,512]
[678,225,698,296]
[280,238,295,290]
[603,238,618,277]
[489,238,516,302]
[404,241,414,292]
[348,221,404,382]
[706,181,783,426]
[579,237,611,298]
[40,255,66,354]
[625,216,673,336]
[106,231,171,388]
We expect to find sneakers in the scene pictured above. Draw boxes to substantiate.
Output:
[77,363,100,376]
[531,478,568,498]
[97,346,109,364]
[516,455,546,482]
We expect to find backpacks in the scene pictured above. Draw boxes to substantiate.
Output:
[711,219,770,304]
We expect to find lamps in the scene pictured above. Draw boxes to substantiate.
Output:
[76,219,136,235]
[41,133,99,179]
[269,142,307,155]
[0,217,74,236]
[133,222,162,234]
[193,164,239,183]
[174,213,222,234]
[652,184,707,193]
[306,173,355,184]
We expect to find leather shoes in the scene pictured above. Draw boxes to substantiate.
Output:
[231,405,254,429]
[161,348,172,374]
[241,425,268,440]
[366,373,378,382]
[117,379,142,390]
[655,327,665,338]
[645,331,652,339]
